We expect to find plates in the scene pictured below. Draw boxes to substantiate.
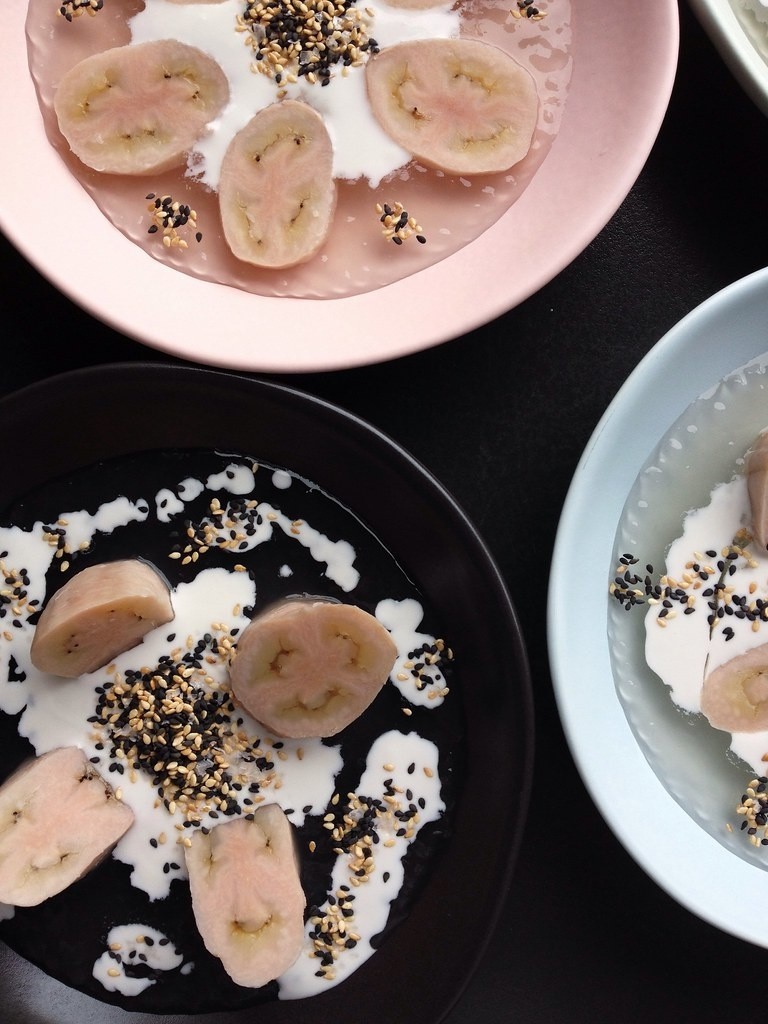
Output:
[0,363,536,1024]
[699,0,768,97]
[548,265,768,953]
[0,0,682,373]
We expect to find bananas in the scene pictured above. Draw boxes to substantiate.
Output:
[53,38,539,268]
[699,430,768,735]
[0,563,397,989]
